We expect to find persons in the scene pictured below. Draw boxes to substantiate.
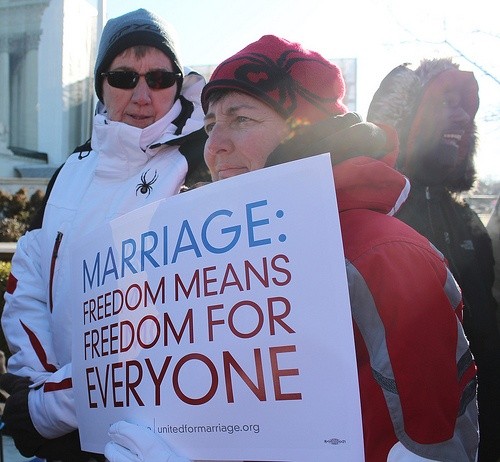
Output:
[0,8,213,462]
[366,57,500,462]
[103,35,481,462]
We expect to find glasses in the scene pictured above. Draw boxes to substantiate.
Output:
[101,69,181,89]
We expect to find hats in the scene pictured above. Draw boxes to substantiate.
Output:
[201,34,349,129]
[94,8,184,105]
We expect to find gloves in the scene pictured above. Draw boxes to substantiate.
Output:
[0,373,50,458]
[104,421,191,462]
